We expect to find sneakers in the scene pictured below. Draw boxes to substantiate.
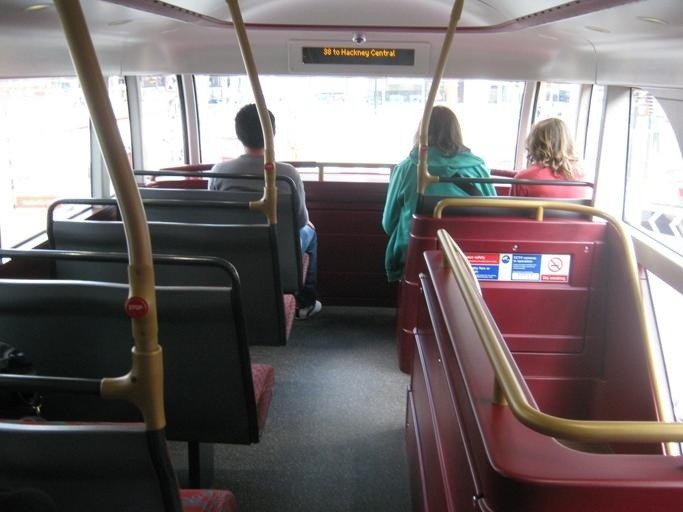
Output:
[294,299,322,320]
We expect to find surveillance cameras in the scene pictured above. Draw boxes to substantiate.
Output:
[352,31,367,46]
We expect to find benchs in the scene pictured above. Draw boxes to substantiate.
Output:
[411,172,594,219]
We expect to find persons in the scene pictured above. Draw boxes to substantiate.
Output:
[380,105,497,284]
[508,118,590,198]
[207,103,323,319]
